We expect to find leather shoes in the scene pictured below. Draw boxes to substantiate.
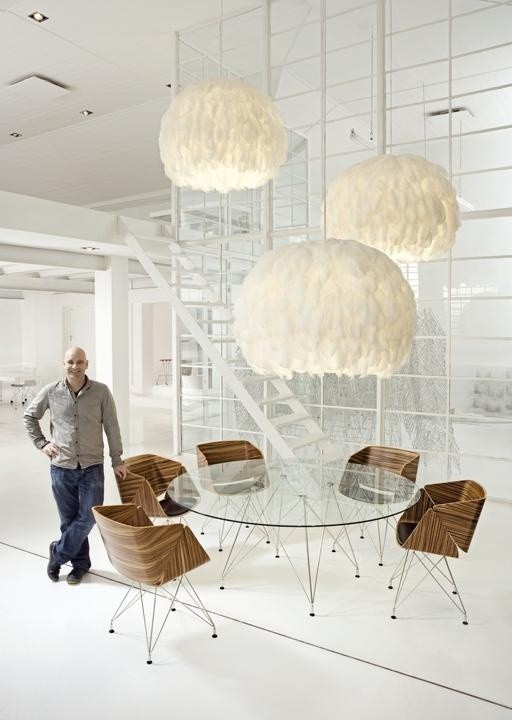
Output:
[47,541,61,582]
[66,563,91,585]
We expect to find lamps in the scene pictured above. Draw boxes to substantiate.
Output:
[320,0,460,263]
[232,0,416,380]
[158,0,289,195]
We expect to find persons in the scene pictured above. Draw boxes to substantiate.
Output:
[22,346,130,584]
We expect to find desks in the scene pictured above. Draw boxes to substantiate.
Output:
[167,458,421,616]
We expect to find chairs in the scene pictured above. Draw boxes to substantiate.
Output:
[91,503,218,664]
[114,454,201,525]
[10,379,38,406]
[196,440,271,552]
[332,446,420,566]
[388,480,487,625]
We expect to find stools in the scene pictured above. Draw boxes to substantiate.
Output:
[156,358,173,386]
